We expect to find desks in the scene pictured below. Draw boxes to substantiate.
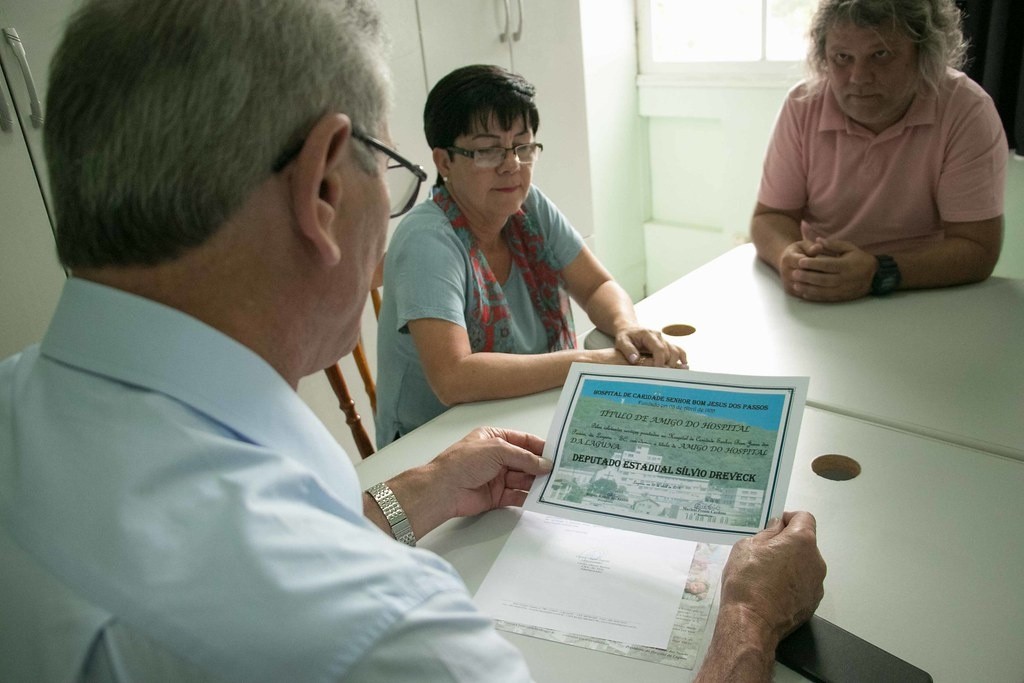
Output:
[583,243,1023,460]
[338,384,1024,683]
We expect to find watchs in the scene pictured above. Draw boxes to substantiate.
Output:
[871,255,901,296]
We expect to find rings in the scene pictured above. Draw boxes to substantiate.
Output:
[639,356,648,366]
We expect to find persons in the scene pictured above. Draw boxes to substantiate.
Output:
[750,0,1008,302]
[683,559,711,600]
[0,0,828,683]
[375,63,690,452]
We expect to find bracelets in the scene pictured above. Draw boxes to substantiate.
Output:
[364,482,416,548]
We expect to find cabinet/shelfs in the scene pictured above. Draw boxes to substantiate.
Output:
[280,0,442,460]
[0,1,82,356]
[416,0,594,239]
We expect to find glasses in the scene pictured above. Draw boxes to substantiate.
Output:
[351,128,428,219]
[446,143,543,168]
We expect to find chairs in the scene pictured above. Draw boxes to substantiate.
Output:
[323,251,385,459]
